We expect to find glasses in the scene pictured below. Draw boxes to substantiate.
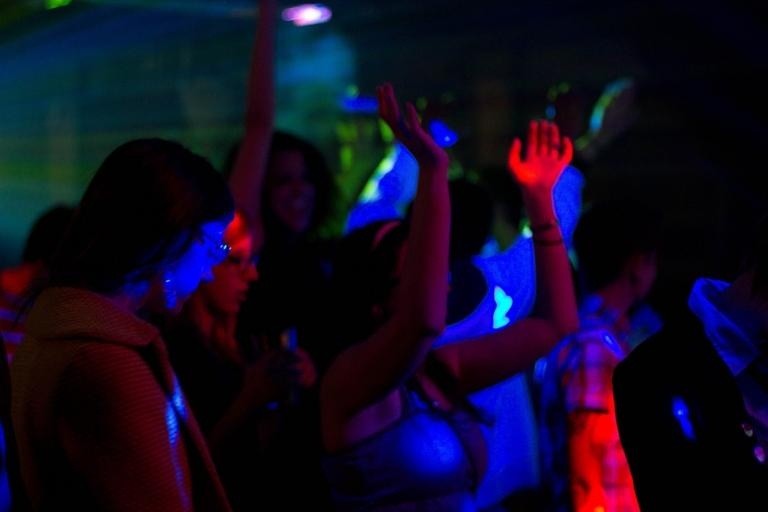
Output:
[204,229,232,263]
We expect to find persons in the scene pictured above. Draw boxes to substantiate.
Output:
[0,0,767,509]
